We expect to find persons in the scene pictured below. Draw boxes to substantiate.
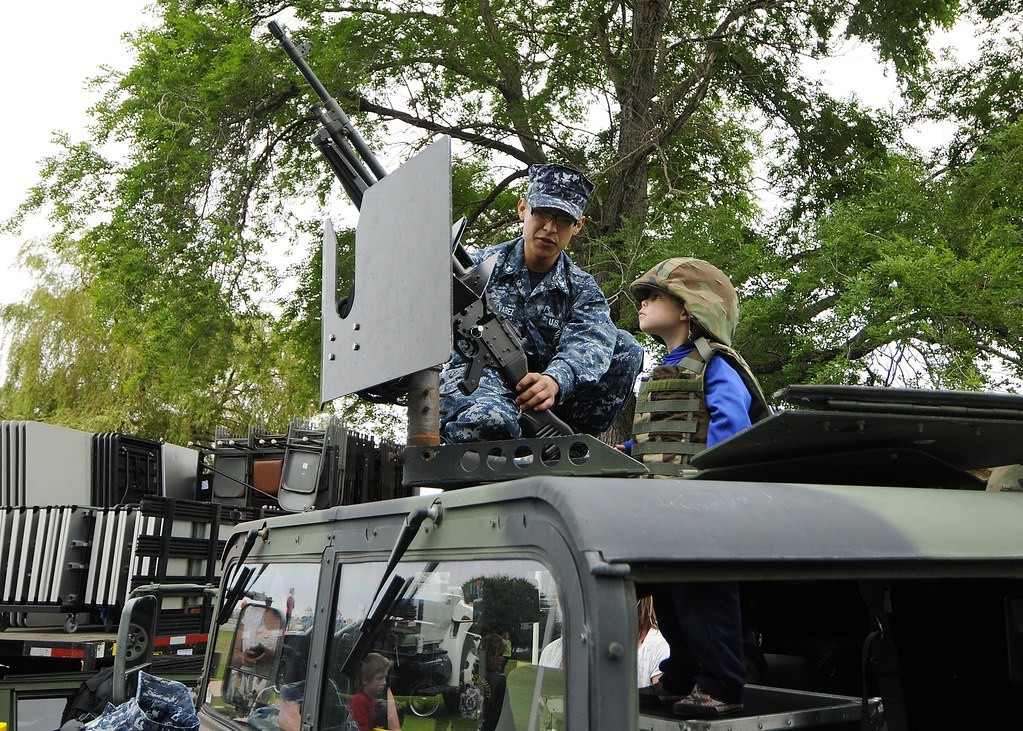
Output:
[499,632,512,675]
[284,588,296,631]
[347,653,391,731]
[637,595,669,689]
[231,606,281,698]
[442,162,643,444]
[652,583,746,716]
[615,258,771,478]
[475,633,506,730]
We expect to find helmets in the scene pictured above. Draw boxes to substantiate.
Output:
[629,257,740,348]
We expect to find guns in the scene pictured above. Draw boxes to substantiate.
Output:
[266,17,585,463]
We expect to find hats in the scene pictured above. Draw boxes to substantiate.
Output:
[525,164,596,220]
[127,669,202,731]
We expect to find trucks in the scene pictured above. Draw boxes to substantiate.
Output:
[58,134,1022,731]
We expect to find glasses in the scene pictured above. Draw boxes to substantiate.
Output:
[527,203,576,230]
